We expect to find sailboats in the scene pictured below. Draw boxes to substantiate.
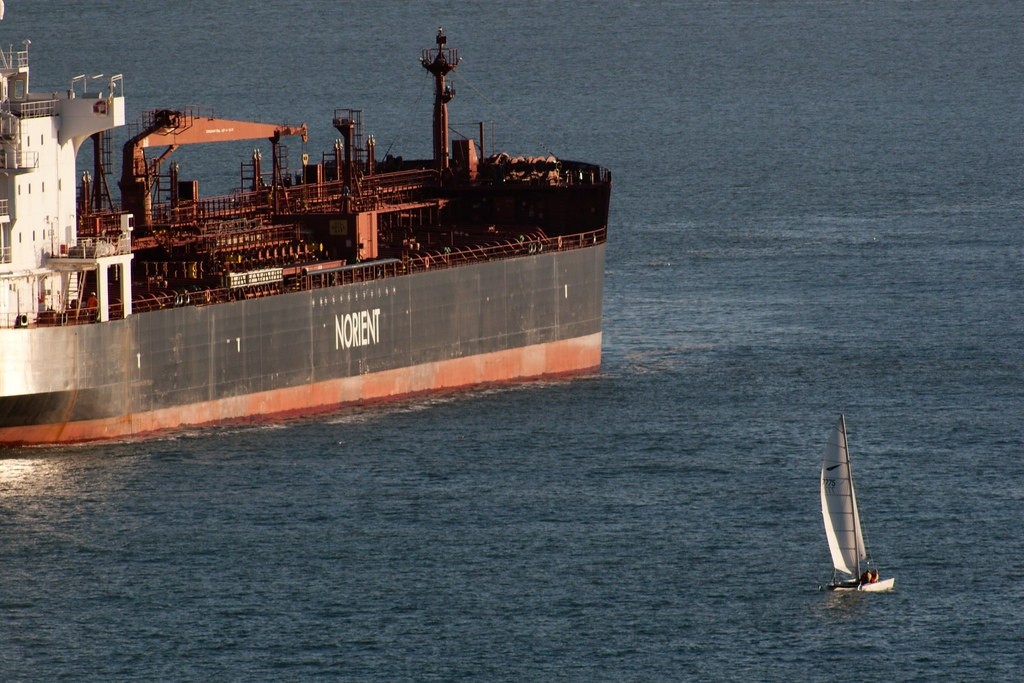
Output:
[819,413,895,592]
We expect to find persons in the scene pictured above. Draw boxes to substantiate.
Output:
[87,292,97,314]
[861,570,872,584]
[871,569,880,583]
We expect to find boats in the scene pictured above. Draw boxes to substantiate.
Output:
[0,0,612,443]
